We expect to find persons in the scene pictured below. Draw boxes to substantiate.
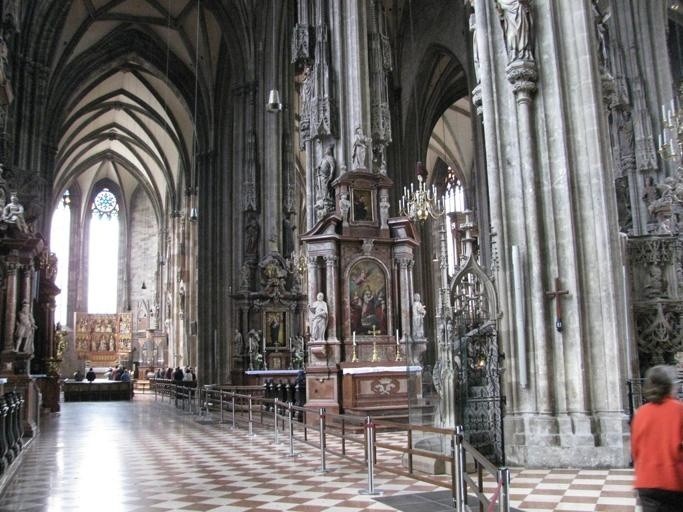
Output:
[309,292,328,341]
[156,366,196,381]
[412,293,426,337]
[350,290,386,335]
[14,299,38,353]
[232,329,243,357]
[631,366,683,512]
[356,195,368,220]
[53,322,69,355]
[86,365,134,381]
[313,145,336,208]
[353,127,372,168]
[3,197,24,231]
[270,316,281,343]
[98,335,115,352]
[642,176,664,222]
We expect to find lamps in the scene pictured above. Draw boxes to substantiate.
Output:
[396,0,447,230]
[261,0,283,113]
